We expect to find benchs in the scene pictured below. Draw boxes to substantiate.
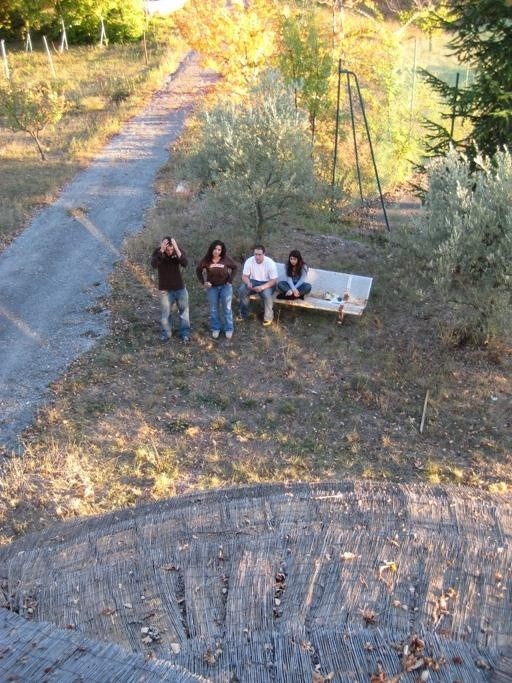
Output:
[249,262,374,326]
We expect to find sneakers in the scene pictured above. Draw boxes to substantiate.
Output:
[225,332,232,339]
[212,331,220,338]
[263,320,272,326]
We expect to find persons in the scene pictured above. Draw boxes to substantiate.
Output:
[235,245,278,327]
[276,250,311,300]
[196,240,237,339]
[151,237,190,343]
